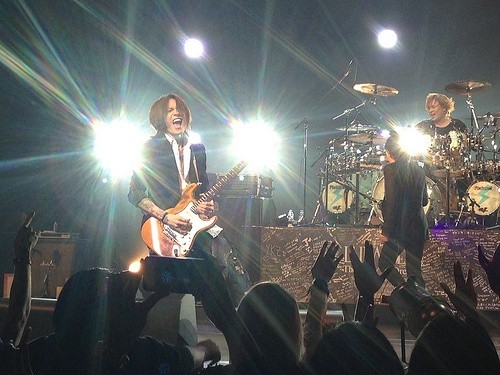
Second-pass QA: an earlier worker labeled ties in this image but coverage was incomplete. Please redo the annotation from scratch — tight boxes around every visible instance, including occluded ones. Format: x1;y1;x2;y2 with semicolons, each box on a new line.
177;140;184;193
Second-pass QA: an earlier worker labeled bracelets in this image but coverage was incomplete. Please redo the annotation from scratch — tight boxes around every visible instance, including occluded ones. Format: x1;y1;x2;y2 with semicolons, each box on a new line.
161;212;169;224
13;255;31;266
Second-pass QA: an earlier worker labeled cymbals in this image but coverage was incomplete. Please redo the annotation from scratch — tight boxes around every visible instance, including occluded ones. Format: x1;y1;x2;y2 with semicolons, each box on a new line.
346;134;386;143
353;83;398;97
445;79;492;95
335;124;380;132
465;111;500;119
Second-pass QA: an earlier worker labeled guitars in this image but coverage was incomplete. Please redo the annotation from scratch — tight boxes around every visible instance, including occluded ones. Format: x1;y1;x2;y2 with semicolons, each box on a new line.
330;177;383;209
140;158;248;257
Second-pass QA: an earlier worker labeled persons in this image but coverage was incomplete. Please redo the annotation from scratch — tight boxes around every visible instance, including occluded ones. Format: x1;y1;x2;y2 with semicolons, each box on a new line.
26;266;222;375
410;93;473;167
0;210;41;375
127;93;250;311
206;234;500;375
376;136;429;292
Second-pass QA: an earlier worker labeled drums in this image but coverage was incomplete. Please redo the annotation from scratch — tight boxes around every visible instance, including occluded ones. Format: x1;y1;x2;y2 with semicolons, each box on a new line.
371;173;452;228
320;180;355;212
463;180;500;216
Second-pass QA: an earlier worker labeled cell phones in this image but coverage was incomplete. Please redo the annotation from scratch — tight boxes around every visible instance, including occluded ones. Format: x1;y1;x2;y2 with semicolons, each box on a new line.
141;256;211;297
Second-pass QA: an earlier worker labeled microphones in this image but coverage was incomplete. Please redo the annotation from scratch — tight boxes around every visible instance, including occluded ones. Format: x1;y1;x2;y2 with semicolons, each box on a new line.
353;59;358;81
